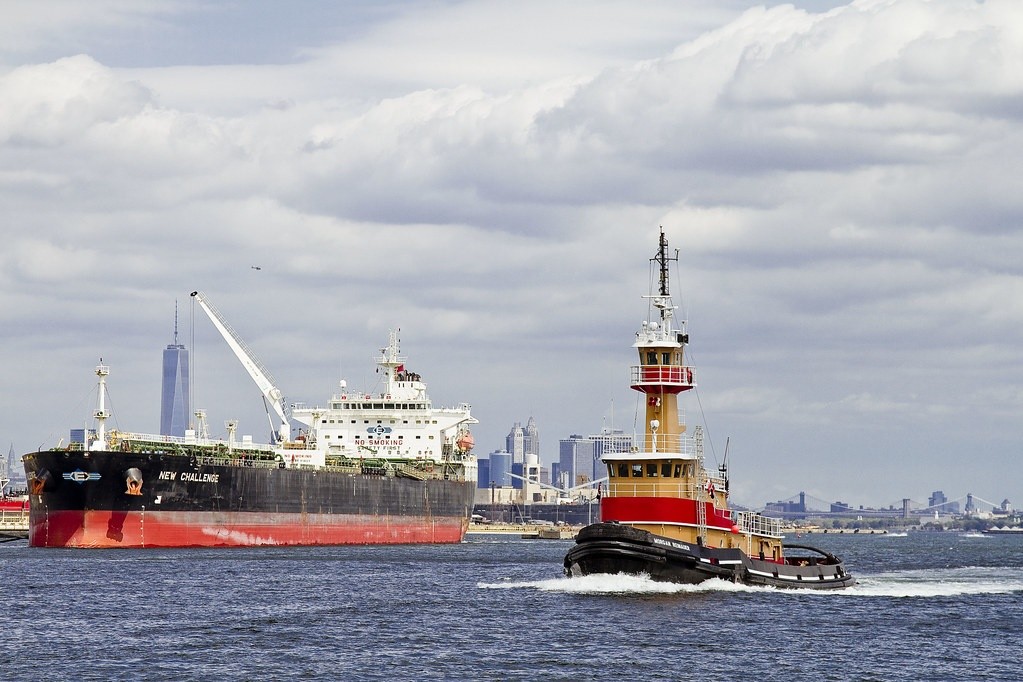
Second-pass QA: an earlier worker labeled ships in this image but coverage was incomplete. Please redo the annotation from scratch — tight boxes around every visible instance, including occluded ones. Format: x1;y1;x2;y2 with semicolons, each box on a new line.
21;291;482;552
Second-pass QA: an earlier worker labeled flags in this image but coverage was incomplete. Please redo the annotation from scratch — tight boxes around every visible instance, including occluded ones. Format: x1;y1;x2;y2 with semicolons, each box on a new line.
397;365;404;372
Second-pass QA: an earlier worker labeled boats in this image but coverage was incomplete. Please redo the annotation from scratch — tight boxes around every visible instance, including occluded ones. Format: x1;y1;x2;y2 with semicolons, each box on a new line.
462;500;588;542
0;476;29;538
563;225;858;588
